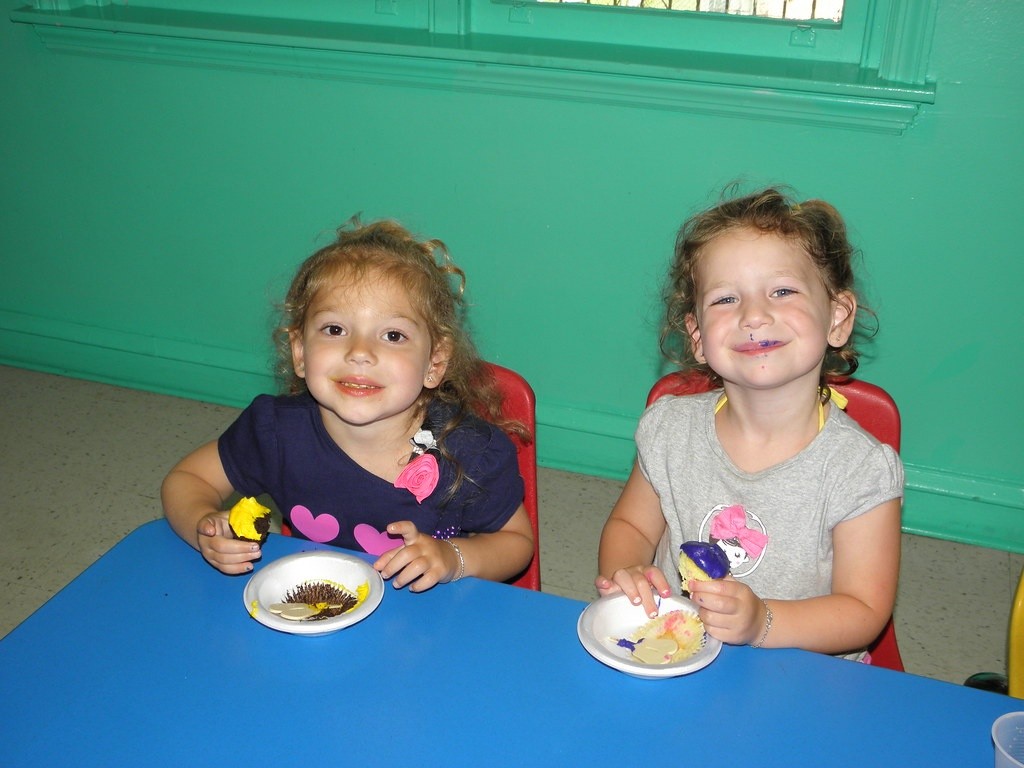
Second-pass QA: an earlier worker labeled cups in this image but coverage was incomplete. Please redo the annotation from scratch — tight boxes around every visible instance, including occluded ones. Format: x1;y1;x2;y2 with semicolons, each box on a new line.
991;711;1024;768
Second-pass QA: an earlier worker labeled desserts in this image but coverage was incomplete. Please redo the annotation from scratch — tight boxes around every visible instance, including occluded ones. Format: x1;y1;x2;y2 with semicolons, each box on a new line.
678;540;730;592
229;496;271;548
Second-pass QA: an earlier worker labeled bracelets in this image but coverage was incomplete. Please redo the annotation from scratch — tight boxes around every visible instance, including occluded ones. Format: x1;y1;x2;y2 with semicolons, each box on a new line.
443;539;464;583
750;598;773;649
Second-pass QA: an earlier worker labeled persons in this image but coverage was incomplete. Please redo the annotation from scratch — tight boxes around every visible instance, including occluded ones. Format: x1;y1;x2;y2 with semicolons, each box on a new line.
590;181;908;667
160;220;536;594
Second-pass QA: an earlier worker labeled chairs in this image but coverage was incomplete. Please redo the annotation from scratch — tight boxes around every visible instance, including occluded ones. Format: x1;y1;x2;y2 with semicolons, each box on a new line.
282;359;542;594
646;364;910;673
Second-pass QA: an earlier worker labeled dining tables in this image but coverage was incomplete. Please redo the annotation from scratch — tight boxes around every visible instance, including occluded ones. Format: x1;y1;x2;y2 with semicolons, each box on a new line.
0;513;1024;767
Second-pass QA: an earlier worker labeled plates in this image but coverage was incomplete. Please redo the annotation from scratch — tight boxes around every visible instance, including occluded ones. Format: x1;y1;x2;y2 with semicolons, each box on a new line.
576;587;723;680
243;550;385;637
